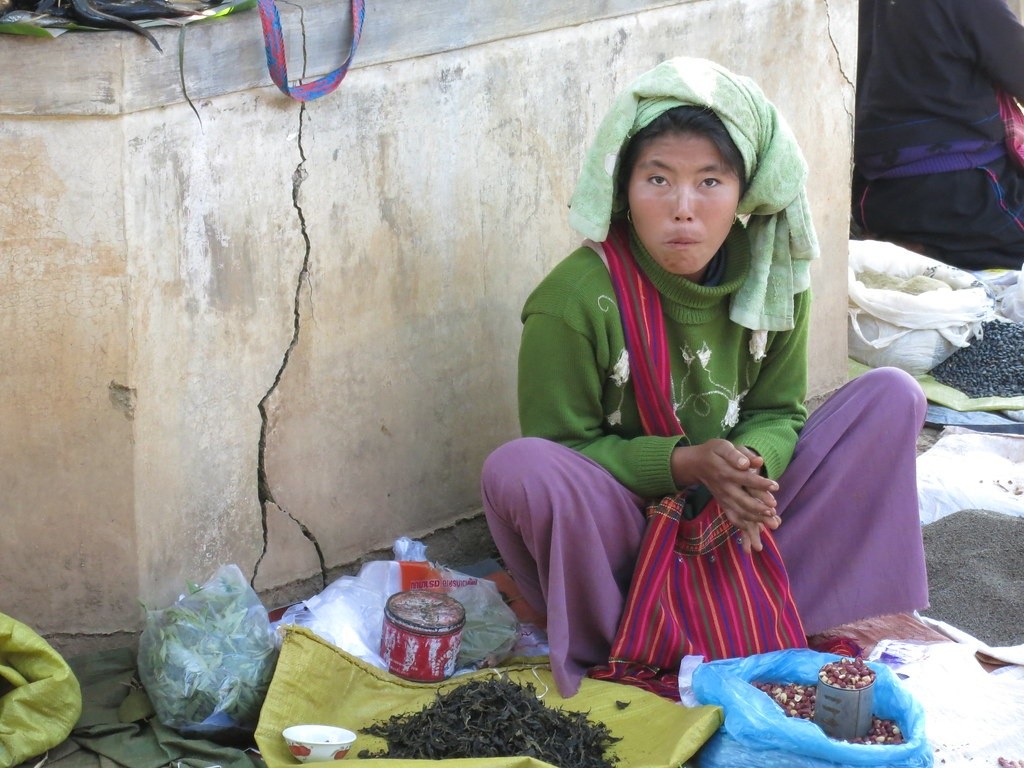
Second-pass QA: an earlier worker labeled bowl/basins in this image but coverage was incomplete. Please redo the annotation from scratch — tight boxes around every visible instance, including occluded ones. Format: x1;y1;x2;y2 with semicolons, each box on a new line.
281;724;356;764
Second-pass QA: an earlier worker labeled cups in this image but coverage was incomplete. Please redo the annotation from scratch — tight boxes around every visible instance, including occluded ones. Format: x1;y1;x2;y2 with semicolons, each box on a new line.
814;662;877;740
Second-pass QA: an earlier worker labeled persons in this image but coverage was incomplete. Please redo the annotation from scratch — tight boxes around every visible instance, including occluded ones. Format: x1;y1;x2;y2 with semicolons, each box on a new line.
857;0;1024;271
480;55;929;700
